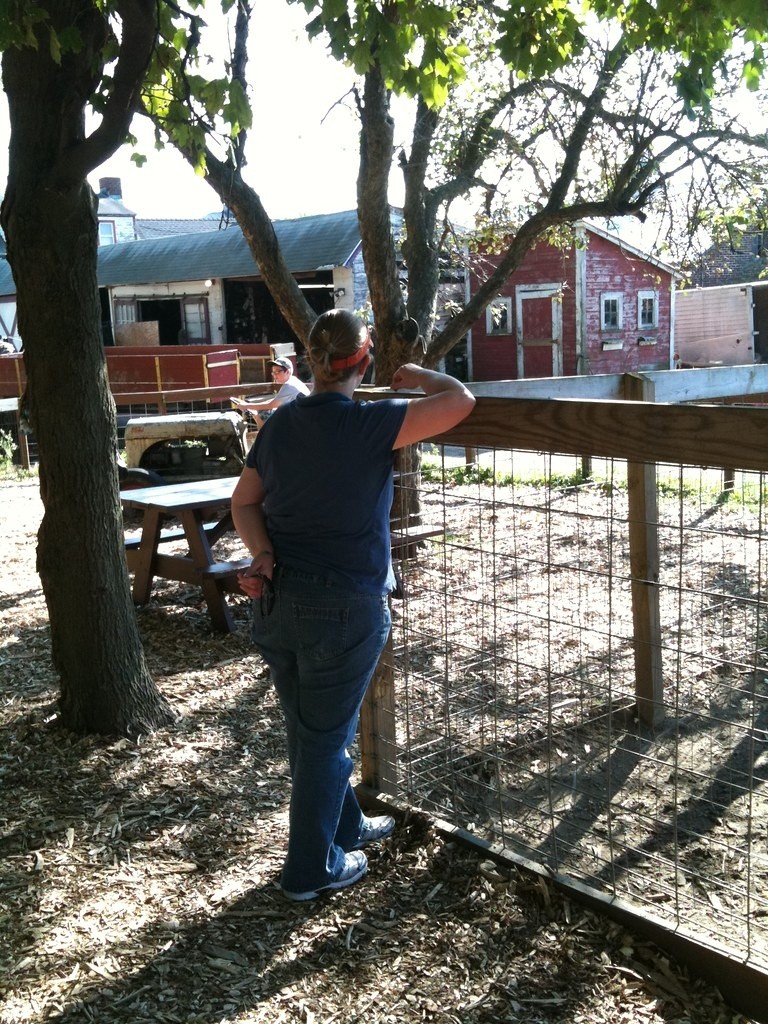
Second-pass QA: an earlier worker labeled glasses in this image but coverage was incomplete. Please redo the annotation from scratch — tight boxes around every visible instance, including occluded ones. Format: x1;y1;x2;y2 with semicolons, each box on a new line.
270;369;284;376
253;573;275;619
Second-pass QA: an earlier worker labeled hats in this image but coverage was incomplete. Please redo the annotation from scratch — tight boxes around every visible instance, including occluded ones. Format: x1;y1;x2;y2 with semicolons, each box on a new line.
266;357;294;376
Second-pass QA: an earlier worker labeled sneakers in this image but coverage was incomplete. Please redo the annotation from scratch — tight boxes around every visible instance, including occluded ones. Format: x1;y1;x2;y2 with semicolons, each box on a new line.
282;850;369;900
352;809;396;849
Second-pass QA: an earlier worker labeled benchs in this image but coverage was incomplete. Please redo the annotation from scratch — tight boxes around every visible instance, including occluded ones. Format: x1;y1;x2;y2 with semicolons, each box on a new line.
204;523;445;600
120;474;242;632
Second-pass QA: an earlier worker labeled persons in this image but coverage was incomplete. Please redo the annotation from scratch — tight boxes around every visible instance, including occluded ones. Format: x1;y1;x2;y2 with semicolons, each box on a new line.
231;306;477;902
235;357;311;430
